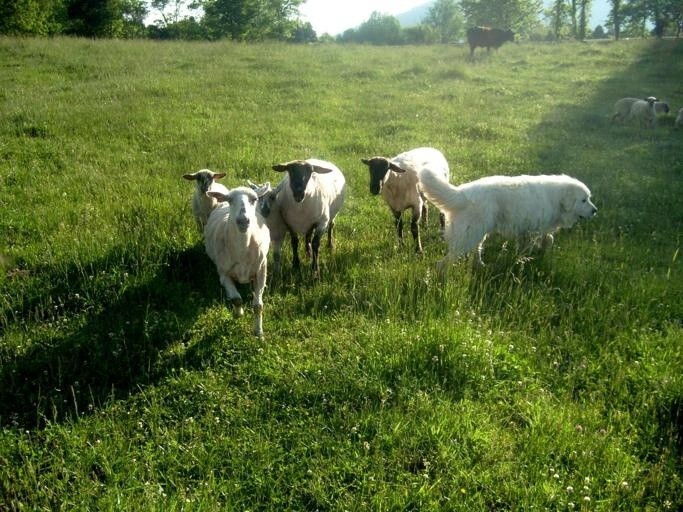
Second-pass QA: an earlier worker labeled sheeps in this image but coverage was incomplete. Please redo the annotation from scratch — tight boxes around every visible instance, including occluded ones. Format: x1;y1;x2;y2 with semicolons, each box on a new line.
361;146;450;262
612;98;683;129
203;182;271;336
271;157;346;282
240;178;286;273
183;169;231;234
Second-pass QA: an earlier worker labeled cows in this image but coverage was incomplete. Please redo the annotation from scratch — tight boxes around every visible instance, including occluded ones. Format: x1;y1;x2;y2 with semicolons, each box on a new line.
466;26;516;57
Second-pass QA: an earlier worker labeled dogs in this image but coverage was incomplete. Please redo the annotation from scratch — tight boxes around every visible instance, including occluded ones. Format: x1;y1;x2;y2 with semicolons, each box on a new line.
417;166;598;277
